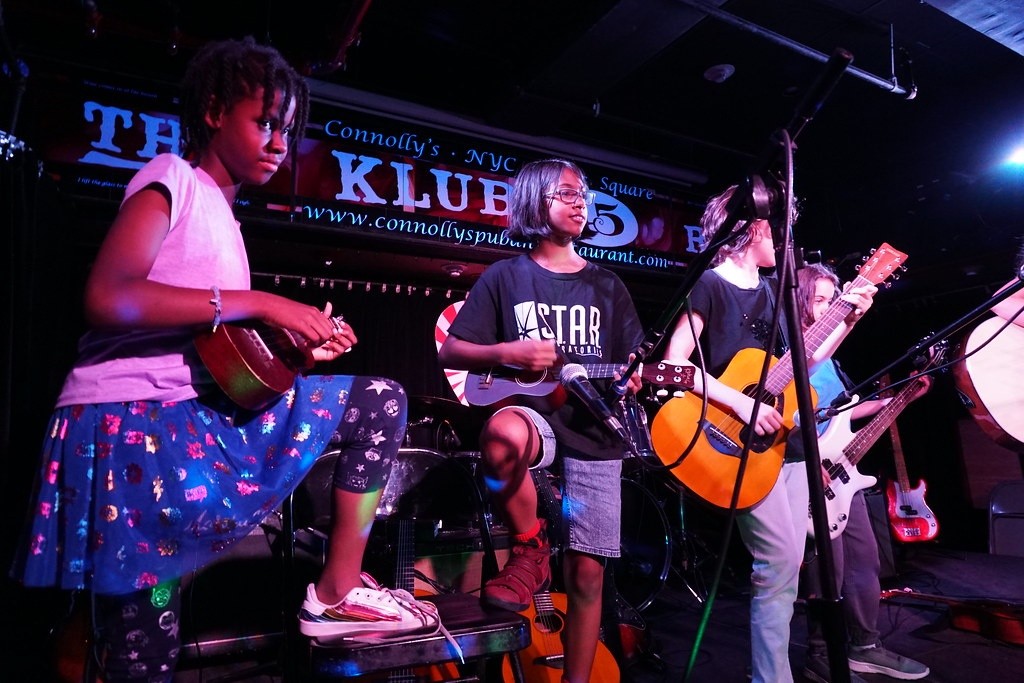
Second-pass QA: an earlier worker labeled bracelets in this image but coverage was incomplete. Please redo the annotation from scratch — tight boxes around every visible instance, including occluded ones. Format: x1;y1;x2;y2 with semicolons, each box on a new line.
208;286;222;332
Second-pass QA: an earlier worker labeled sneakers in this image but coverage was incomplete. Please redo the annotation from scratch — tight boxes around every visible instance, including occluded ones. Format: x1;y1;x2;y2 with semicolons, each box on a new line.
485;539;552;611
803;652;867;683
848;639;930;680
300;572;465;664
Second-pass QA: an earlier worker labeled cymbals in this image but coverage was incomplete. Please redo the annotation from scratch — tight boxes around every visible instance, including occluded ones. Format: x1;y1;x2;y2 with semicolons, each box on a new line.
412;392;489;429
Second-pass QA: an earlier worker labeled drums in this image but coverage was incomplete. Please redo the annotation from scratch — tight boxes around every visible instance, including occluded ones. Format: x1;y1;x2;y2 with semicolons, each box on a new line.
567;465;711;622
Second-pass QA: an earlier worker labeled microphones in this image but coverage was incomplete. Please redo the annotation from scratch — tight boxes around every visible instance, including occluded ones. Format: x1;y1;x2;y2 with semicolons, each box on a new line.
559;362;629;443
794;407;838;427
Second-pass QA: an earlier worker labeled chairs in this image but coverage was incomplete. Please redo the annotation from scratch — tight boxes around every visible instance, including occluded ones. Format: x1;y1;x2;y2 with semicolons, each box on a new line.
287;444;536;683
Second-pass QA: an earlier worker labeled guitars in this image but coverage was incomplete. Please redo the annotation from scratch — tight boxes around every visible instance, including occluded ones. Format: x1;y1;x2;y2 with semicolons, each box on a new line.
808;323;959;540
880;587;1024;645
195;306;348;416
488;446;623;683
950;312;1024;452
464;345;697;419
873;373;942;545
359;486;469;683
643;239;909;513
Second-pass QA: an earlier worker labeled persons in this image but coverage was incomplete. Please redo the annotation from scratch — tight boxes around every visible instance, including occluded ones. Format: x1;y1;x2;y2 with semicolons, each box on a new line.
665;176;812;683
792;265;931;680
22;37;442;683
437;157;645;683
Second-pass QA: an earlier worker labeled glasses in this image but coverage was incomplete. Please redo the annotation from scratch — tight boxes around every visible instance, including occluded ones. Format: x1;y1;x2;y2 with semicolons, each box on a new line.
544;188;596;206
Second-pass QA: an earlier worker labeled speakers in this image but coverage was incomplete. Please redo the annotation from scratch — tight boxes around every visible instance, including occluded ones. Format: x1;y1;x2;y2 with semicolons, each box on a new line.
864;494;895;580
